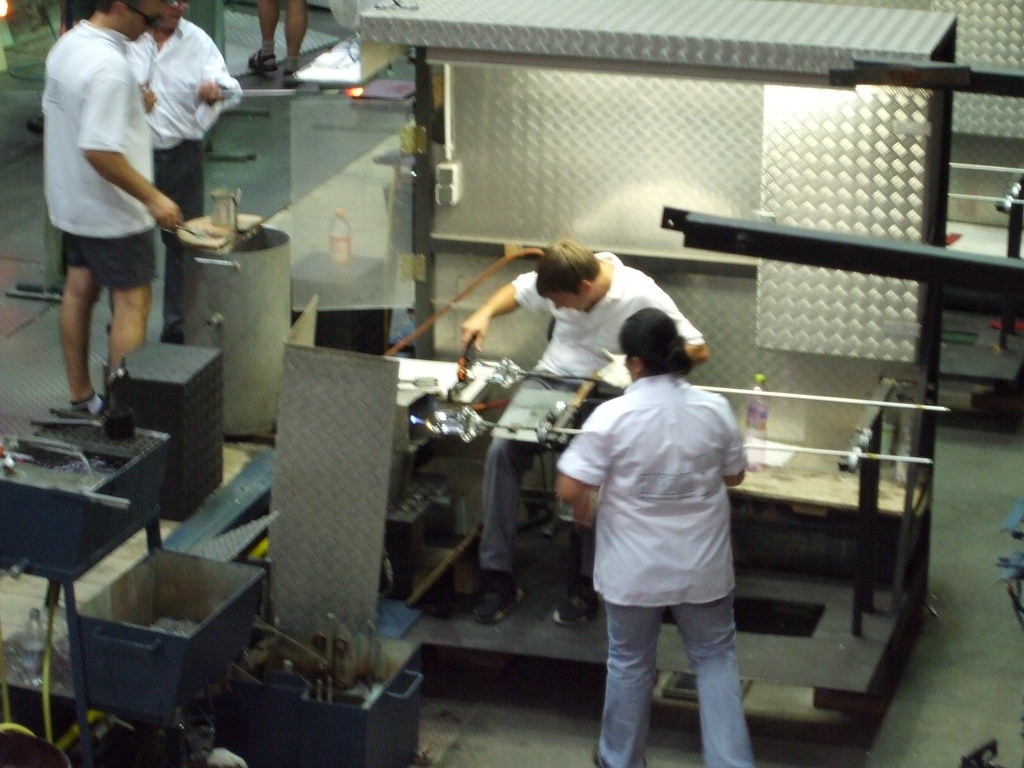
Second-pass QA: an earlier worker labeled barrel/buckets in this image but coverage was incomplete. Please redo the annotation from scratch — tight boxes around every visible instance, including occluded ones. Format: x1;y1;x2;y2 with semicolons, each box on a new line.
164;226;292;435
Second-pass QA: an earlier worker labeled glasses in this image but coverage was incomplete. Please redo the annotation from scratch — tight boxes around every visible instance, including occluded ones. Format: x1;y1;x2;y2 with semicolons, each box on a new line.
127;5;159;28
162;0;187;9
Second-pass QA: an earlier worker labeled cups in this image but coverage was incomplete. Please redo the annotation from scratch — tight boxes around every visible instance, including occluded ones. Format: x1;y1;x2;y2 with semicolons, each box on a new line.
212;188;239;227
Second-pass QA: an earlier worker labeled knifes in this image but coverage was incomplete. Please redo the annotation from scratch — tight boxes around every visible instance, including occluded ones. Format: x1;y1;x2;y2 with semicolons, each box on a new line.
177;222;210;239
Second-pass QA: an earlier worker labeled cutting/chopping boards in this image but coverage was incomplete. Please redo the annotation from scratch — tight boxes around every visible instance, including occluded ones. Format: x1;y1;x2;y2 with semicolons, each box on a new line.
176;213;262;252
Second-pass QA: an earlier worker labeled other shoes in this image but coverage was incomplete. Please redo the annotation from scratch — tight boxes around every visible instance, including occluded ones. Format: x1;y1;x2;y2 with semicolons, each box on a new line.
70;393;108;417
161;333;184;344
594;749;646;768
552;576;599;627
474;571;523;623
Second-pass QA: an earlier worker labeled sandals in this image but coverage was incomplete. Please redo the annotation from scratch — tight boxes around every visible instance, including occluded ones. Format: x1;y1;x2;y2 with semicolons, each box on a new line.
249;49;277;72
283;68;298;87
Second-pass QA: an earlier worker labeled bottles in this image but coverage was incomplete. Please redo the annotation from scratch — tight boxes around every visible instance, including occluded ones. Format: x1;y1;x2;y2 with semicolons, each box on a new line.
328;208;350;266
880;378;899;455
20;609;47;686
744;374;768;471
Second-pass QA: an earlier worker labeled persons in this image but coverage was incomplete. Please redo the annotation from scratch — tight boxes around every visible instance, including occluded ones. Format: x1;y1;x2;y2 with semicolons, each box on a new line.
555;308;756;768
249;1;309;86
42;0;184;413
107;0;242;344
461;241;709;625
